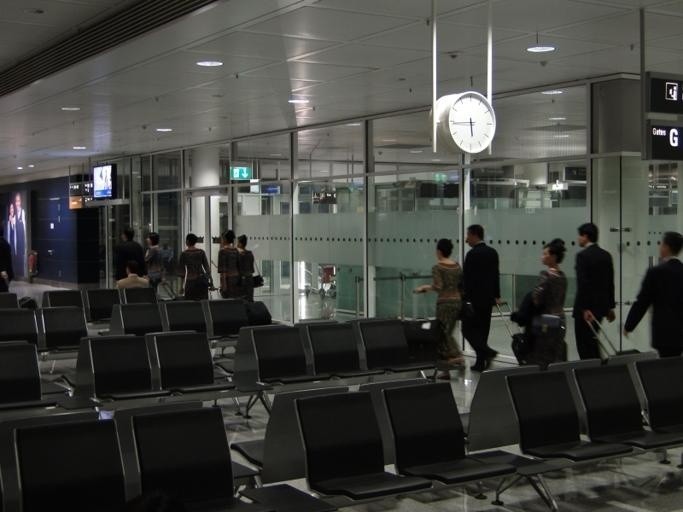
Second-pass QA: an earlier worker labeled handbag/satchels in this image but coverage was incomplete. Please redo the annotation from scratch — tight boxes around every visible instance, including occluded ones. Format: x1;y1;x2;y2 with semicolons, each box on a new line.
531;313;561;338
252;274;264;289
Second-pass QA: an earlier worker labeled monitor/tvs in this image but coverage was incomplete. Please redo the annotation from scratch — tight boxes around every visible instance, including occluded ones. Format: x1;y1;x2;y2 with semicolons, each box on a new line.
92;163;117;199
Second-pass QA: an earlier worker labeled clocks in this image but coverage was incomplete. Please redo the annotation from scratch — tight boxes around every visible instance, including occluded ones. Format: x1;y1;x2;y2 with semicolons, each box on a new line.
429;0;496;158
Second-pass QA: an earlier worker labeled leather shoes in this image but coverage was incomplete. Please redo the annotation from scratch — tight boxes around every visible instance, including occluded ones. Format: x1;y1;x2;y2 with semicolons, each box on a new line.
437;374;449;379
470;350;499;372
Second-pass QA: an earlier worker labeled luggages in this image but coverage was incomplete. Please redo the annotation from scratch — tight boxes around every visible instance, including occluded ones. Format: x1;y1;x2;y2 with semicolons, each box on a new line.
245;300;272;326
161;282;185;300
496;301;530;366
586;315;641;356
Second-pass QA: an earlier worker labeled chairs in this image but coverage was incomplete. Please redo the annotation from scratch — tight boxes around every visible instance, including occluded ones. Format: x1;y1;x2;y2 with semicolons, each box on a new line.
0;286;250;374
0;316;439;421
1;352;683;511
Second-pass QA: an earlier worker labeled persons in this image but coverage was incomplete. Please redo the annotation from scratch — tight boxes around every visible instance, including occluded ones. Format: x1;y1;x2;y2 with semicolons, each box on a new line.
114;226;255;302
6;191;27;277
0;225;15;292
